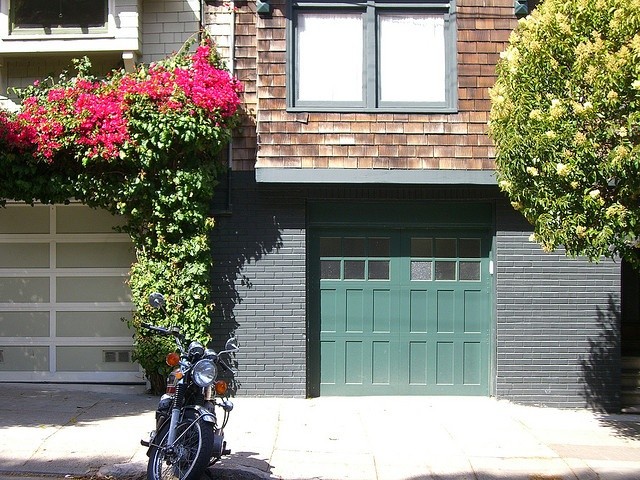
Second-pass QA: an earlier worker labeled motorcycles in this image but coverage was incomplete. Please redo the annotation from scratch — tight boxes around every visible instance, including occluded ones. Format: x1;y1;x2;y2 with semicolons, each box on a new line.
140;292;240;479
